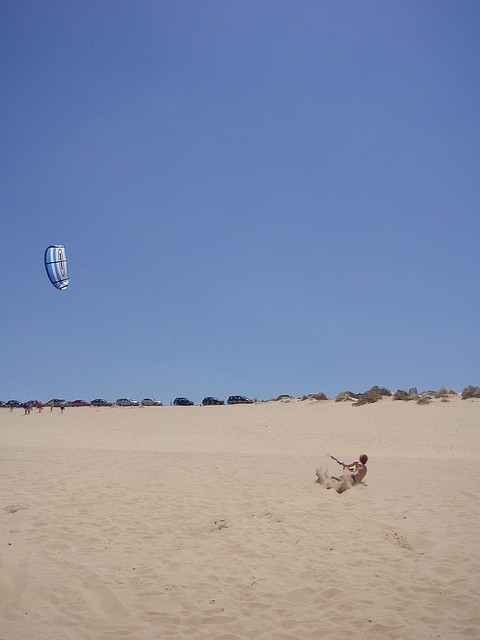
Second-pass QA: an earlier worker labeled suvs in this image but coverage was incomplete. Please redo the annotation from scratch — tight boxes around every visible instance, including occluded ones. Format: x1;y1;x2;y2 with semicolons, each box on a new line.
141;398;162;406
227;396;252;404
173;398;194;406
116;398;139;406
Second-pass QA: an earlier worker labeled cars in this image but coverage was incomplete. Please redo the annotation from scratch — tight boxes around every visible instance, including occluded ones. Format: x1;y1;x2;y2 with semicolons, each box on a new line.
71;400;90;407
46;399;66;407
6;400;22;407
23;400;44;407
202;397;222;405
91;399;113;406
0;401;6;406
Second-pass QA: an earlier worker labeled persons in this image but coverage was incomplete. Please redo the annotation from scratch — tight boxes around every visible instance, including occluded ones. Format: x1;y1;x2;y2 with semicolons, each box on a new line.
334;454;368;492
